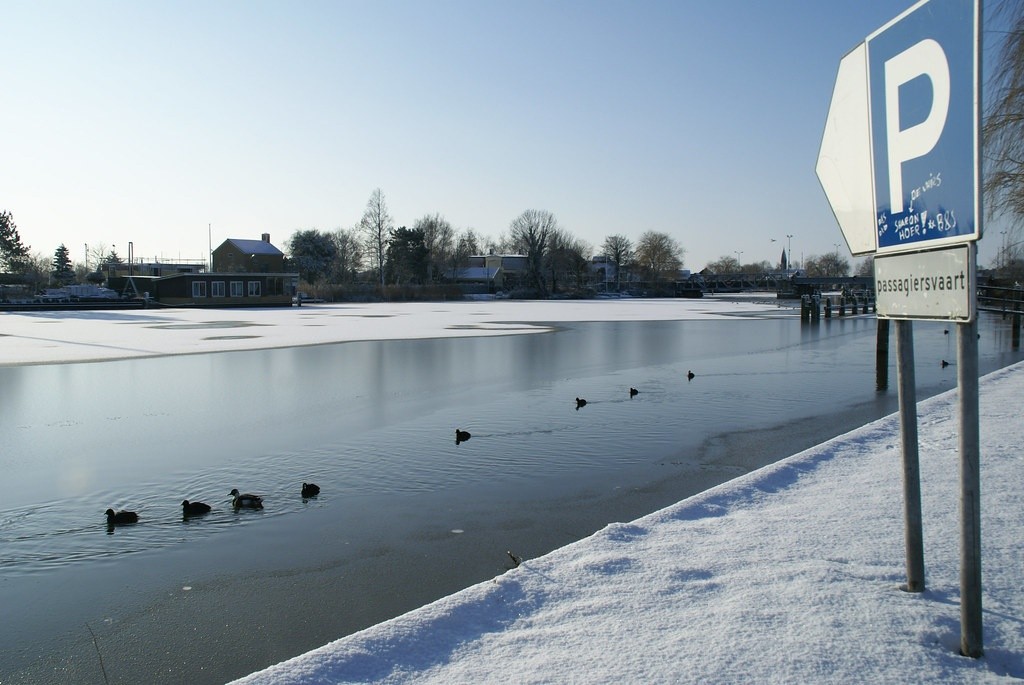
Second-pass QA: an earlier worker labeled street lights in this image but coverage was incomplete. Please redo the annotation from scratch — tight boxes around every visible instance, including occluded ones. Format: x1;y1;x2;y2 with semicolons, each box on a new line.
834;243;840;277
1000;231;1007;268
734;250;743;273
787;234;793;279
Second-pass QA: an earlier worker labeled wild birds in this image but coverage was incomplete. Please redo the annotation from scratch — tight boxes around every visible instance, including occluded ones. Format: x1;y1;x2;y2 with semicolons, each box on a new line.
227;489;264;508
943;329;949;335
455;438;470;445
455;429;471;438
301;482;320;498
688;369;695;376
940;359;949;365
180;500;211;516
104;509;138;524
630;393;638;399
630;387;638;393
575;396;587;404
575;404;586;411
688;376;694;382
